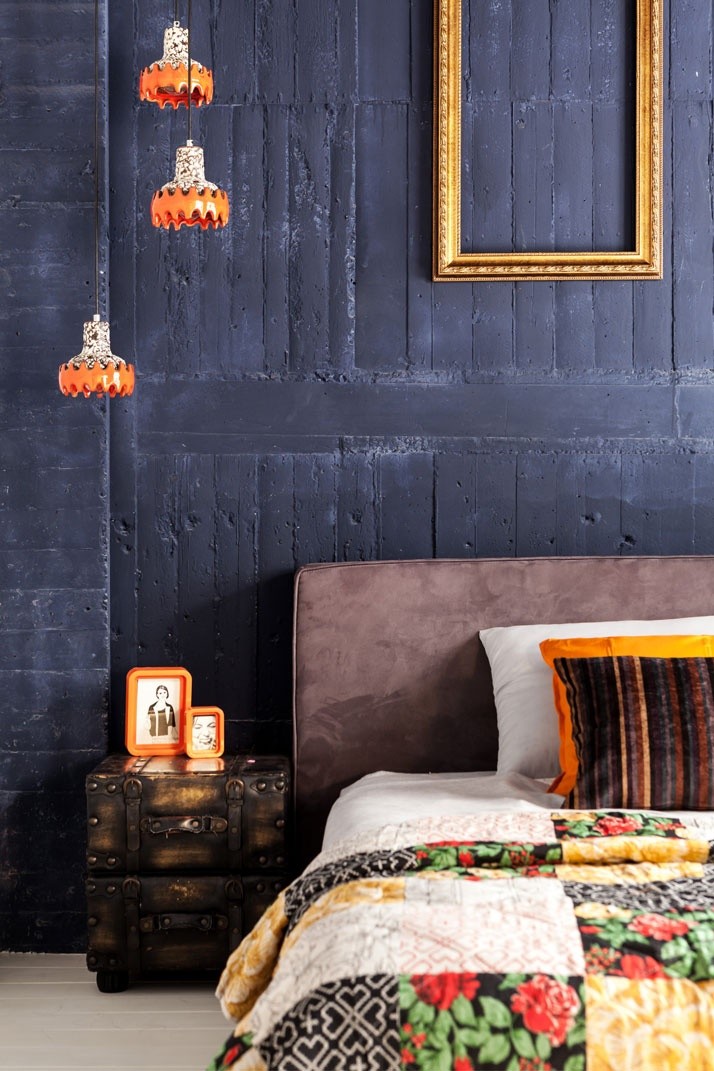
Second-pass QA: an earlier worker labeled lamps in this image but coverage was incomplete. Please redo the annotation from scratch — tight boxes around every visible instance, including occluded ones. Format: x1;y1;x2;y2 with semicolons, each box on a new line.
139;0;213;111
150;0;231;231
57;1;136;400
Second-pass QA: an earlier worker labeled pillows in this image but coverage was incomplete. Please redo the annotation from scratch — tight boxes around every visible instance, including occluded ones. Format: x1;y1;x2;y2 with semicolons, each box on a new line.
476;614;714;780
538;633;714;801
552;654;714;812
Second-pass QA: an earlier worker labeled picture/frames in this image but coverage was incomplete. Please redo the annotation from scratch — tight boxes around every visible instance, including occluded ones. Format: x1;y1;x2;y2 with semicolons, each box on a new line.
125;666;193;757
183;706;226;758
430;0;665;283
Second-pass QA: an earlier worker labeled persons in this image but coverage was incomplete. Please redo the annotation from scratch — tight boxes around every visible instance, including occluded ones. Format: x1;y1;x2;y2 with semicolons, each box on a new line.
142;685;178;744
193;716;216;750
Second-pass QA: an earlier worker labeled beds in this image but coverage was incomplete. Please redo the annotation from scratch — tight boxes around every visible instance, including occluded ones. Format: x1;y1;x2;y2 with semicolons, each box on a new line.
209;552;714;1071
